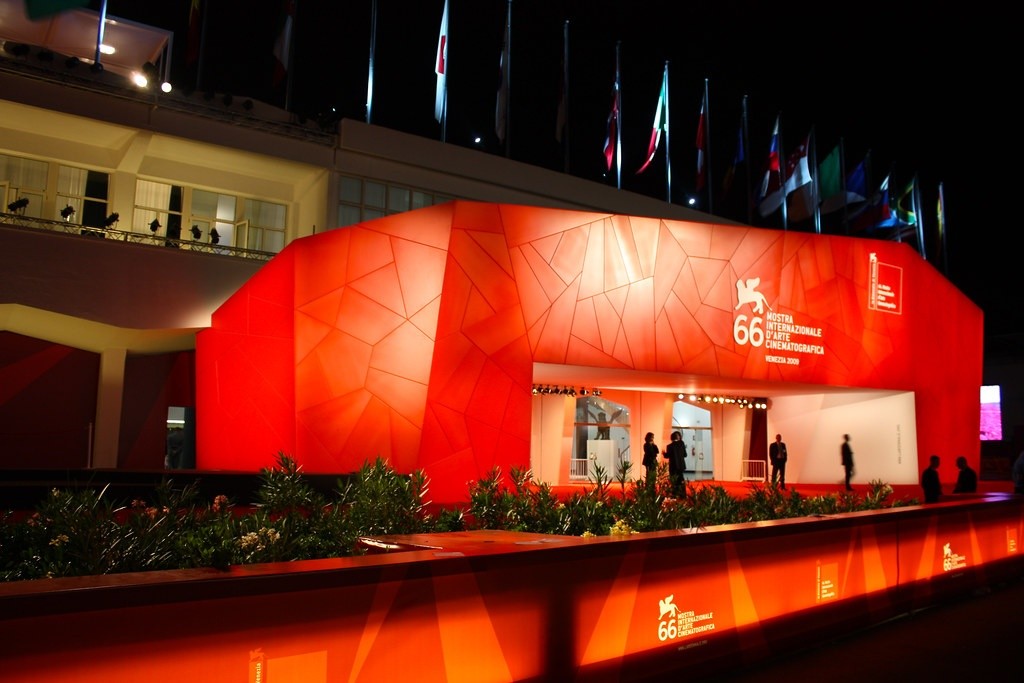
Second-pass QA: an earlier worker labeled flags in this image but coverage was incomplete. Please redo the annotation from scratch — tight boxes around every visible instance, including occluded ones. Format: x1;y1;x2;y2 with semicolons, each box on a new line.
187;0;200;64
695;97;944;263
496;19;508;143
602;73;619;172
434;0;449;122
635;70;665;174
555;60;565;143
23;0;91;20
272;0;296;87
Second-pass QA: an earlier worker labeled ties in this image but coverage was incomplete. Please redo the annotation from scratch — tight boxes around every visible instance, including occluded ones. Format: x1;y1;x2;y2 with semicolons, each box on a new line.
778;443;782;456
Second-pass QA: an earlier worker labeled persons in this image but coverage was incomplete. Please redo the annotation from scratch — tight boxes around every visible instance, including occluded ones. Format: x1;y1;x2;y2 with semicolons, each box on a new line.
662;432;687;499
953;458;977;493
1012;452;1024;493
841;435;853;490
642;432;659;489
921;455;943;503
166;427;184;469
769;434;787;489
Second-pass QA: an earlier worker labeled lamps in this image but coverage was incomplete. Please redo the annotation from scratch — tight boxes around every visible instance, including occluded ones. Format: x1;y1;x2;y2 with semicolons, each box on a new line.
8;198;30;213
208;227;222;243
533;384;601;397
678;393;768;409
189;224;202;240
149;218;162;233
61;205;75;220
103;212;119;228
